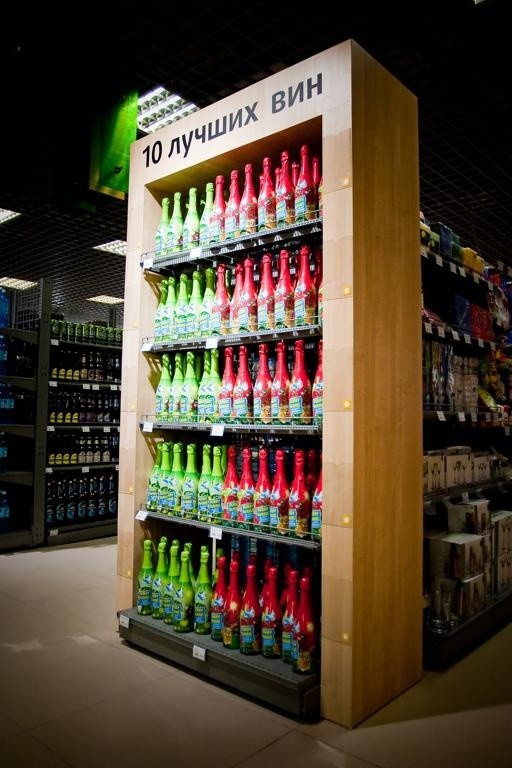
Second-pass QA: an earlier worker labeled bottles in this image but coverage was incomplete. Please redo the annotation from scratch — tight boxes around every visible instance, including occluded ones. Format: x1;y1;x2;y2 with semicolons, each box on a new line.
182;188;199;250
102;437;110;461
51;350;58;378
88;325;95;338
73;351;79;379
237;258;258;332
0;432;8;472
211;264;231;334
174;274;189;339
210;557;227;642
292;576;315;675
163;546;180;624
156;443;171;513
305;449;316;493
71;395;79;422
50;319;59;336
155;198;170;257
239;164;257;237
311;339;323;424
211;549;224;588
62;435;70;464
81;324;88;338
293;246;316;326
74;323;81;337
59;321;66;337
95;326;102;339
79;394;85;422
224;170;238;241
208;446;225;523
210;176;224;243
70;435;78;463
168;442;185;517
102;328;108;339
57;394;63;422
97;394;104;422
199;183;214;246
254;448;272;533
318;173;323;218
289;450;310;539
161;536;169;572
0;335;8;375
104;395;111;423
161;276;177;342
67;322;74;337
313;252;321;286
114;328;121;341
166;192;182;253
240;565;261;655
172;540;181;577
86;437;94;463
186;271;203;338
88;352;94;380
180;352;198;422
221;448;240;526
168;354;184;422
261;568;280;658
275;151;294;227
233;346;254;424
154;354;171;421
5;384;14;423
95;353;102;379
229;263;243;332
152;542;168;618
270;448;290;536
86;395;93;421
274;250;294;328
312;155;319;186
197;350;211;423
288;340;311;423
80;354;87;379
198;445;212;522
257;158;276;230
254;344;272;424
66;350;73;378
291;162;299;187
137;540;154;615
153;279;168;341
50;394;56;423
199;268;216;337
317;279;324;325
281;571;299;664
237;447;256;530
64;394;71;422
204;348;221;423
112;395;119;423
270;342;291;424
48;435;55;464
257;254;275;329
55;435;62;464
294;144;314;221
94;437;100;462
223;562;241;648
145;441;164;511
221;445;227;479
181;444;200;519
108;327;114;340
174;551;195;633
194;553;213;634
78;437;85;463
185;543;196;587
0;383;4;424
58;350;66;378
114;355;120;382
0;491;10;534
218;347;235;423
310;454;322;540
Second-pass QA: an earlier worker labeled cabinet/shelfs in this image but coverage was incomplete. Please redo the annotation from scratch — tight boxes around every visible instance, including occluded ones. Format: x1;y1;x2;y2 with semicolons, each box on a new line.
117;38;424;729
419;220;512;673
49;278;123;546
0;277;48;550
